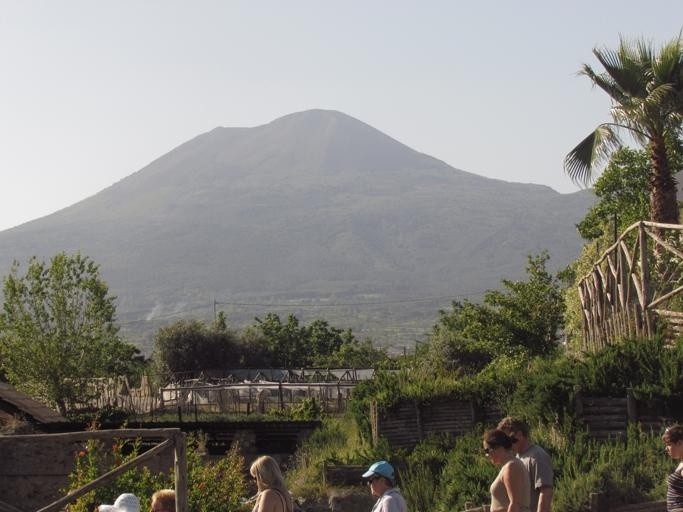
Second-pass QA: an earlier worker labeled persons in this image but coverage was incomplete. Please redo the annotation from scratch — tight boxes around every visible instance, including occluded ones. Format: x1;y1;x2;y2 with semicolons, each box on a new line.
248;454;293;511
661;423;683;511
149;489;176;511
494;415;555;511
482;429;534;512
359;459;408;512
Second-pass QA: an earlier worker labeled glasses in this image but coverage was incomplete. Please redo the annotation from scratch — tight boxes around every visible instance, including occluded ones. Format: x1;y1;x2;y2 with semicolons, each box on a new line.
366;477;380;485
483;447;493;453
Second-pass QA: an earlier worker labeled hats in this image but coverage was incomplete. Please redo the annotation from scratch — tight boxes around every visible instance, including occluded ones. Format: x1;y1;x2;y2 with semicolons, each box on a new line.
360;460;394;479
